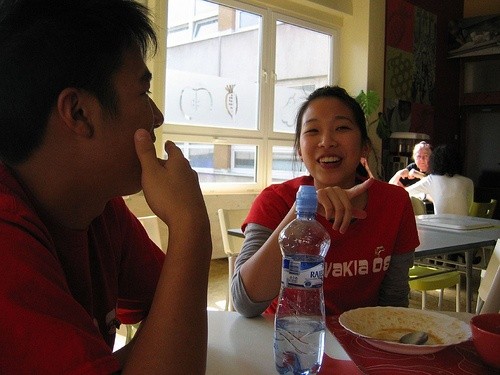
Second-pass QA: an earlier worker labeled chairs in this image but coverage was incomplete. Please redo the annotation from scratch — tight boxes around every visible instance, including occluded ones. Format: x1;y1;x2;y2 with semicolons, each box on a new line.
123;200;500;346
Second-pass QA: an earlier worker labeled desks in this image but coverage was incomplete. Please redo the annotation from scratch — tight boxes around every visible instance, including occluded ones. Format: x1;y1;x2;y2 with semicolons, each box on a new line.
206;309;500;375
227;212;500;313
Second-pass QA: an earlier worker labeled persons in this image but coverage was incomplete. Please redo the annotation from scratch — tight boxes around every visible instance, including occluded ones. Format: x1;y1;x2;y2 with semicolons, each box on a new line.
0;0;221;375
231;86;420;318
390;142;434;216
401;147;481;275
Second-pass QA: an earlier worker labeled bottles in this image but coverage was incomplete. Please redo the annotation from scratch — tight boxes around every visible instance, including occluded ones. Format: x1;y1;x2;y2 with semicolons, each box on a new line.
272;185;331;375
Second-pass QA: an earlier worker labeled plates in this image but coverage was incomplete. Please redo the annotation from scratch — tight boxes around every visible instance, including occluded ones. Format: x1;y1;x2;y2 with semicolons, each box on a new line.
338;307;472;356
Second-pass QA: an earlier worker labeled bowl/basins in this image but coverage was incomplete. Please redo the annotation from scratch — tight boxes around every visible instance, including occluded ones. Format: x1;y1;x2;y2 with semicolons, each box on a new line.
470;312;500;370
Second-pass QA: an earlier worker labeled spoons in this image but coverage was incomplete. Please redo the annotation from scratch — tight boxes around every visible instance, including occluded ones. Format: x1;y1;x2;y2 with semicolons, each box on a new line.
359;332;429;345
